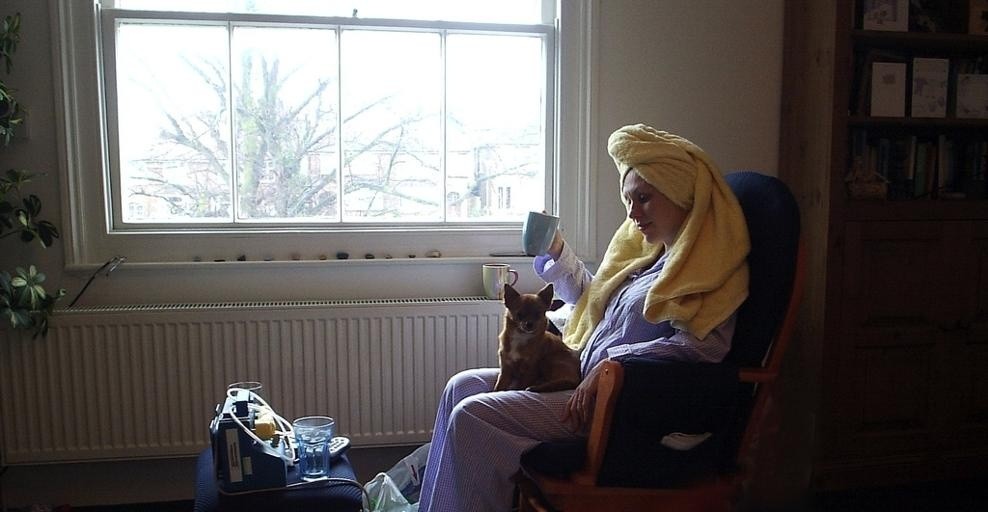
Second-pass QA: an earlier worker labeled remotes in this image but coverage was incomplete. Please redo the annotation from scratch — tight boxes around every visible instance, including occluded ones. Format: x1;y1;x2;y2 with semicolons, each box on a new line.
328;437;351;461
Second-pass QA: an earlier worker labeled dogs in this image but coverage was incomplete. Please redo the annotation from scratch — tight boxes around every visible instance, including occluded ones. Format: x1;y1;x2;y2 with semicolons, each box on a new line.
493;283;581;393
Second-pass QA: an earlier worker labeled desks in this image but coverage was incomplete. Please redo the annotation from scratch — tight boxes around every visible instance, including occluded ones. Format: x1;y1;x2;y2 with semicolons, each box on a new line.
194;445;365;511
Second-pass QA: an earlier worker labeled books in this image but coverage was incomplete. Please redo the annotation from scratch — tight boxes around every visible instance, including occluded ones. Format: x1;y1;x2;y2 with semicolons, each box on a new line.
853;1;987;201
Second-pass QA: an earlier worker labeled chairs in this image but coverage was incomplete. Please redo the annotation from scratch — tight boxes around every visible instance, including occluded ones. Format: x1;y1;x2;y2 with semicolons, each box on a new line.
512;171;807;512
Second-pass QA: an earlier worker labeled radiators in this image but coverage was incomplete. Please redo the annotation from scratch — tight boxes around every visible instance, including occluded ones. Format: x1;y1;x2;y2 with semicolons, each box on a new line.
0;295;510;466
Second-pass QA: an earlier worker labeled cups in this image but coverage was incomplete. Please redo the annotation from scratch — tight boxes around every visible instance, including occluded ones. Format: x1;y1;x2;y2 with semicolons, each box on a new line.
483;262;520;302
291;414;334;482
228;381;262;397
521;211;561;258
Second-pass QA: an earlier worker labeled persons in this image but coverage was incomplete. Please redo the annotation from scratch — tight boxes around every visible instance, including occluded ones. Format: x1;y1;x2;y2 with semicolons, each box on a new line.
417;122;753;512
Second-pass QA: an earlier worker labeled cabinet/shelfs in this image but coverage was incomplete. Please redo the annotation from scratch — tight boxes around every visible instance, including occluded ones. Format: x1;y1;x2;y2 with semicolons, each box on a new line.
776;0;988;501
